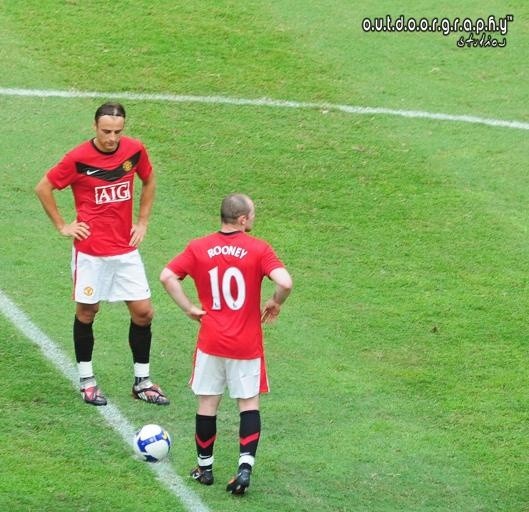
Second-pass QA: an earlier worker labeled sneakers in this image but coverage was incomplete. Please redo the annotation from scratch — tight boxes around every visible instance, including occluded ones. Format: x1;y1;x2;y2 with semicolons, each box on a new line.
226;468;250;495
133;384;170;404
80;384;107;405
191;467;213;485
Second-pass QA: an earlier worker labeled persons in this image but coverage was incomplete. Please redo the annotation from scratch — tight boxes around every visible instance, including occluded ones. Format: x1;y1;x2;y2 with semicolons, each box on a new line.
35;104;169;406
161;194;292;494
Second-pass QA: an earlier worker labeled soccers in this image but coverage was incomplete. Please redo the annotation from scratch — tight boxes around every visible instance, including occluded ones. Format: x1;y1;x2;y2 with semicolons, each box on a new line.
134;424;170;463
